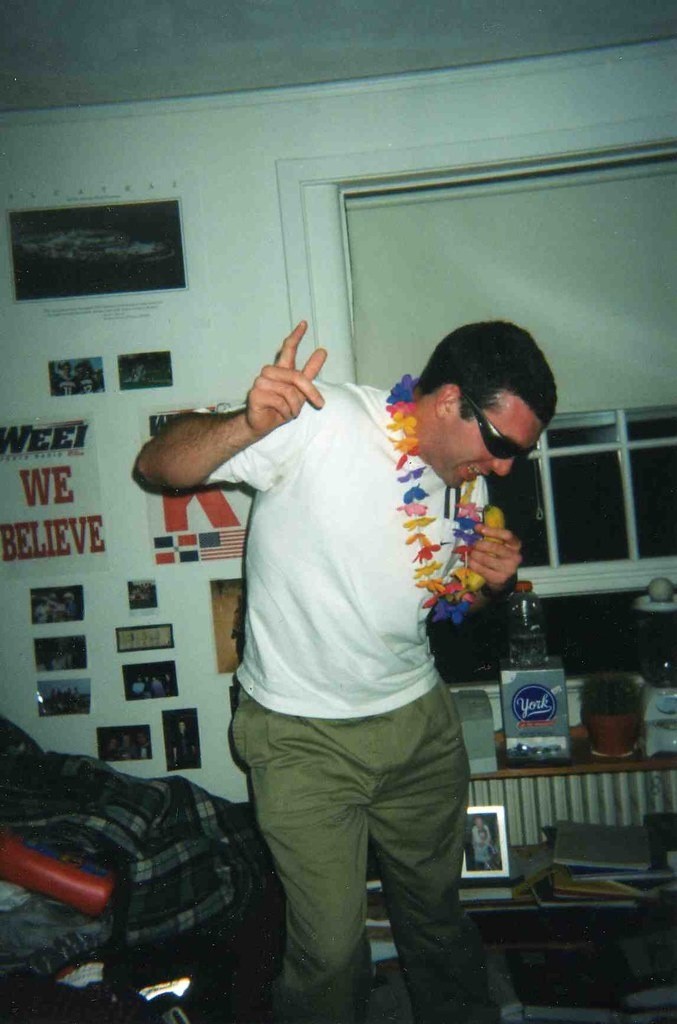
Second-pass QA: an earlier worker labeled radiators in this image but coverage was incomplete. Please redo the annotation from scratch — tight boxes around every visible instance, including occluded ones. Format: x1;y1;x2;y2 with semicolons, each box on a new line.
465;769;677;847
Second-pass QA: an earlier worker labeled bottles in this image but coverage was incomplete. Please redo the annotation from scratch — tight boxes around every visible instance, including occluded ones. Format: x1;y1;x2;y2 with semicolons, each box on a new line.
508;580;550;669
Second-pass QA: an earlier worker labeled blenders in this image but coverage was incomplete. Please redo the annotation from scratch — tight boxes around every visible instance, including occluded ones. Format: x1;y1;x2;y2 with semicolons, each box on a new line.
633;579;677;760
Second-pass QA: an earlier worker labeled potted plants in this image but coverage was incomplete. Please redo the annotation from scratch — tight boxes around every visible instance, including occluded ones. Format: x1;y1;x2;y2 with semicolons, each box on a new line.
581;682;642;756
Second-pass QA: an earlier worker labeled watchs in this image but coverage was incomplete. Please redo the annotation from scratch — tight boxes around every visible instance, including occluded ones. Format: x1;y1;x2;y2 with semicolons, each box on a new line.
482;574;518;601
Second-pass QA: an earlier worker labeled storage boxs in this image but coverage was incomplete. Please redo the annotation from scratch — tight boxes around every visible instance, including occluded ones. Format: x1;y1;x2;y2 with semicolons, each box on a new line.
499;656;574;765
452;689;498;775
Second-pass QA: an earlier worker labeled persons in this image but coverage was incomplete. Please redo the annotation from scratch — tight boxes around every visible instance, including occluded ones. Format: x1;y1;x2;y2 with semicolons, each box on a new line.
54;360;98;396
133;672;176;699
132;583;153;601
50;687;80;715
108;732;152;761
50;643;73;671
171;718;196;770
137;320;558;1024
33;592;78;624
472;815;496;870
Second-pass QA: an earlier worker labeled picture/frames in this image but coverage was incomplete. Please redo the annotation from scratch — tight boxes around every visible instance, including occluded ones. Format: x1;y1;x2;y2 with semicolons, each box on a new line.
460;805;510;878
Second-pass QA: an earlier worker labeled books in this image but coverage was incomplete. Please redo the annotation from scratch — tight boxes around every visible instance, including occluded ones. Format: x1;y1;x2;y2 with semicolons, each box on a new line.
514;813;677;907
496;962;677;1024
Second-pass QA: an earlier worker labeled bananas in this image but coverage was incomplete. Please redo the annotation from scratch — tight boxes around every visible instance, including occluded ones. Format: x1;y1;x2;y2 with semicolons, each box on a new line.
465;504;505;592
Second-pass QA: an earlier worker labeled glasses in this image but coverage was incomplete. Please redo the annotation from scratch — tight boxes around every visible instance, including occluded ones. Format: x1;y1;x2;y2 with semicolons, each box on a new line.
461;390;537;461
